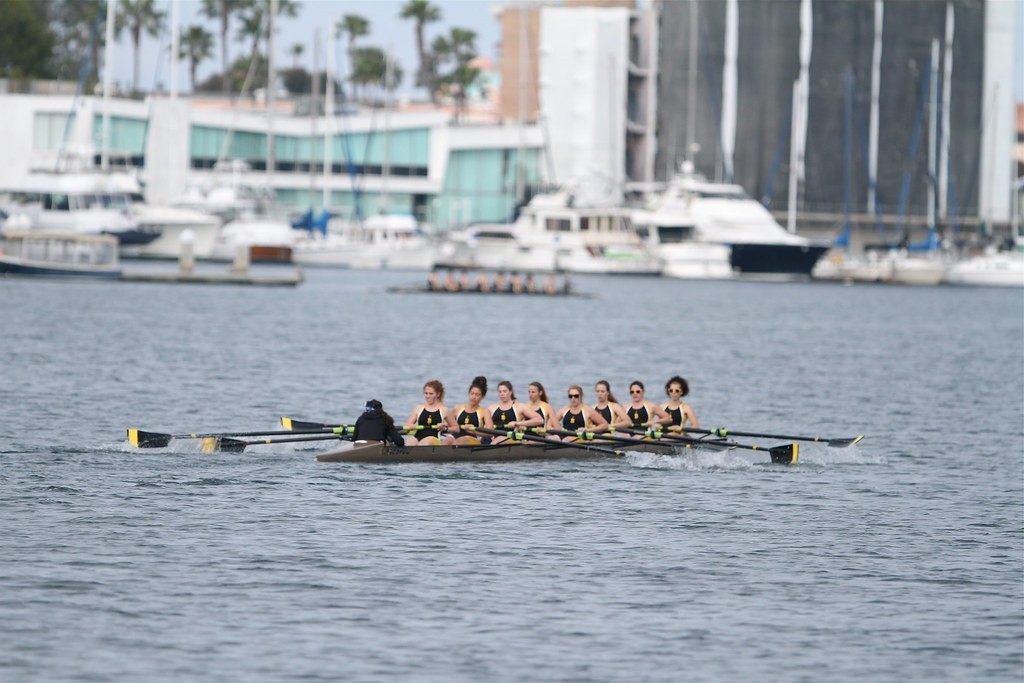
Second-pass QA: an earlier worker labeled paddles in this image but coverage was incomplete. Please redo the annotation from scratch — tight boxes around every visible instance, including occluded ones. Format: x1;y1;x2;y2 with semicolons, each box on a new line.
458;425;627;458
660;425;864;448
127;428;356;455
201;434;355;453
607;425;799;465
281;416;447;431
494;425;692;449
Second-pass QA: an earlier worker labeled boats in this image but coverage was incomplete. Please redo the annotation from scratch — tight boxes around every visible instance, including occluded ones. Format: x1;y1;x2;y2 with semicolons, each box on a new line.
0;230;124;281
316;437;736;464
123;269;306;289
388;284;580;297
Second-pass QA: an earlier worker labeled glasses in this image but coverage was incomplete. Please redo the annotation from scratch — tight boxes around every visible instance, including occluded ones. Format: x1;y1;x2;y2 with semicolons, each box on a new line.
669;388;682;392
569;394;580;398
630;390;642;394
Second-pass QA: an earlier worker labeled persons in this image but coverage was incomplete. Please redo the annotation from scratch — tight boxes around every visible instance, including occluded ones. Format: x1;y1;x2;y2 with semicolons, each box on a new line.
480;381;544;445
427;267;555;295
351;398;404;447
547;384;608;443
519;382;562;444
588;380;633;442
616;380;673;439
402;380;460;445
653;376;699;441
442;377;494;444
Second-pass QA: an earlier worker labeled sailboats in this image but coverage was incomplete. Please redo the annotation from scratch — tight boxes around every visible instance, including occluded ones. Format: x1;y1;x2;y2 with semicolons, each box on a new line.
1;0;1023;286
